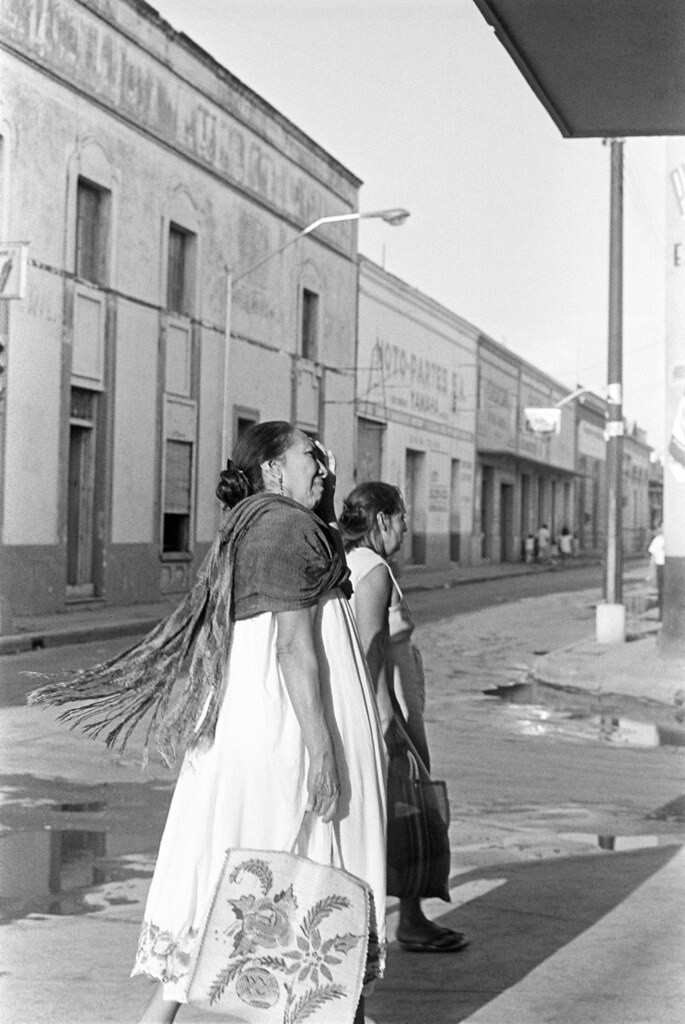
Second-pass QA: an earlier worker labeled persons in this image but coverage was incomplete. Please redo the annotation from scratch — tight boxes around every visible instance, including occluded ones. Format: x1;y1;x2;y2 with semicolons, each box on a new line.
525;522;576;567
333;483;474;954
129;421;389;1023
647;521;664;620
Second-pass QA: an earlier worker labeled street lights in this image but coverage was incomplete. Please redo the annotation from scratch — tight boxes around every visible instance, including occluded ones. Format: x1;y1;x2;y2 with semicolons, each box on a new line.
220;209;410;472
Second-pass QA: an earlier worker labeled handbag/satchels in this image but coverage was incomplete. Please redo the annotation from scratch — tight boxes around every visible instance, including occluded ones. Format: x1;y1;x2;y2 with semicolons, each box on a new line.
376;718;453;903
185;806;370;1024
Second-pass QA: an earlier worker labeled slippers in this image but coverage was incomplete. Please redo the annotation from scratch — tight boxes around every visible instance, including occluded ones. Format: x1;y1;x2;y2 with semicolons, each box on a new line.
398;921;468;952
